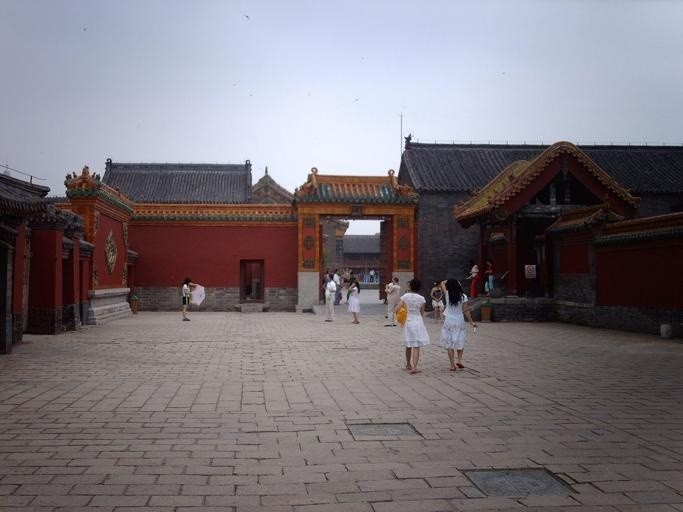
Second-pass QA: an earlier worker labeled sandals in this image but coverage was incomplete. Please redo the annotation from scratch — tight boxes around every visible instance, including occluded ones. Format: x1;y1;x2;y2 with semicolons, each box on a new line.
406;364;422;374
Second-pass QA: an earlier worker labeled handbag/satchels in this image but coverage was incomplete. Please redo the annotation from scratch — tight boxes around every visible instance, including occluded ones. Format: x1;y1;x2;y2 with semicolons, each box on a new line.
396;303;407;324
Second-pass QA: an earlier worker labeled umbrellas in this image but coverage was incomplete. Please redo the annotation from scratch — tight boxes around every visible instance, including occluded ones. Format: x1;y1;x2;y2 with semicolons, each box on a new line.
190;284;206;307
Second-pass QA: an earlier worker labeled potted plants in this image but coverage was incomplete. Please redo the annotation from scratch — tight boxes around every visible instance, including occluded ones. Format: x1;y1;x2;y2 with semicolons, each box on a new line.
129;293;139;315
480;299;493;323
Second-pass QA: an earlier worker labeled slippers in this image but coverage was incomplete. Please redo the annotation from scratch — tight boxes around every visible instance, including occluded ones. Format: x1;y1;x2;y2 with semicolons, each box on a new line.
450;363;464;371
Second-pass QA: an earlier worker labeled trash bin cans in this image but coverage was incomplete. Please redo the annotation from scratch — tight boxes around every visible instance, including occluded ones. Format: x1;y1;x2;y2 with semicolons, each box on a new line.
129;295;138;313
481;300;492;322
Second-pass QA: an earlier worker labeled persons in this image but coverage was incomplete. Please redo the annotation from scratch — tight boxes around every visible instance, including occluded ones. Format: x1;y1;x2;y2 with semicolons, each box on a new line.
322;267;361;324
369;268;376;282
395;279;477;374
182;277;198;321
383;277;401;327
466;258;496;299
